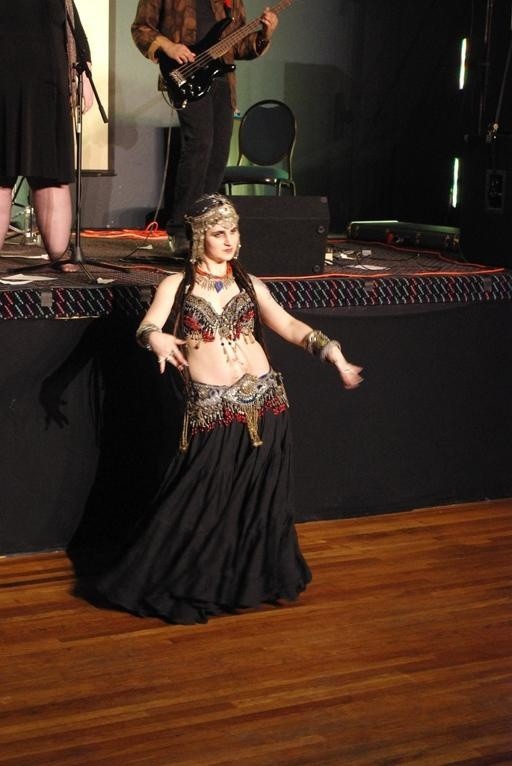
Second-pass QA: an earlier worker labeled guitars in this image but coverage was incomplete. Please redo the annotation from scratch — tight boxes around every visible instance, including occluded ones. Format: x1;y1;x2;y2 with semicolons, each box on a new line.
158;0;293;102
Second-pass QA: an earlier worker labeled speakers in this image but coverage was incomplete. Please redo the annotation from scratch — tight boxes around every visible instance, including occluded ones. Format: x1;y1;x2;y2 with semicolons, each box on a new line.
200;193;331;280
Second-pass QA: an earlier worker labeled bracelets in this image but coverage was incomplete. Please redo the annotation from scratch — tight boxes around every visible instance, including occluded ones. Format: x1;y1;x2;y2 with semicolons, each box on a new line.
303;330;341;361
136;321;162;350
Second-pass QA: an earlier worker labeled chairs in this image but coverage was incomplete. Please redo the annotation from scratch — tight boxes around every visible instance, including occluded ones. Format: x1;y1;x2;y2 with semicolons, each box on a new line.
223;99;297;197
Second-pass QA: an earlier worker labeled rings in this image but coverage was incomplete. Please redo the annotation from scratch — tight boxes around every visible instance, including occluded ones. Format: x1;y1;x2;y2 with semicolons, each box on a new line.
158;351;176;362
342;366;351;373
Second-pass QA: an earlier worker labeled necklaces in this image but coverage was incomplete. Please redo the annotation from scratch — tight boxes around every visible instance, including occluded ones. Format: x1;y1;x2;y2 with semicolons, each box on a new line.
192;272;236;292
194;263;234;278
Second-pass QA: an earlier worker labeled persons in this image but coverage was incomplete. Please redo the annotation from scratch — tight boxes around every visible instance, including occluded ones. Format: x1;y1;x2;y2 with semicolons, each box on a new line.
128;0;280;265
63;190;364;627
1;0;96;276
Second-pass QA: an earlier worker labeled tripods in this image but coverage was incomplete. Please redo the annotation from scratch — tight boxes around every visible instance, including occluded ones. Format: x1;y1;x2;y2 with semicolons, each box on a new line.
8;70;130;283
